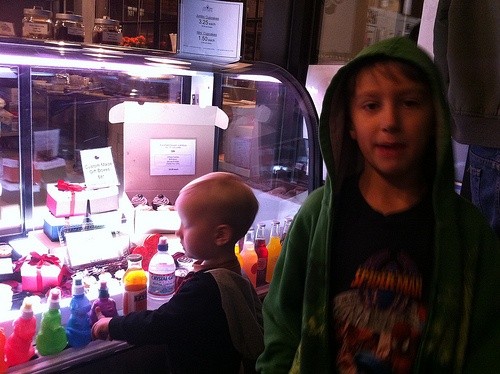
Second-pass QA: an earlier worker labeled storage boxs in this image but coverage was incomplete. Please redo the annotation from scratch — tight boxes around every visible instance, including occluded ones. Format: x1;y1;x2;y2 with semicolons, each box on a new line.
41;101;229;241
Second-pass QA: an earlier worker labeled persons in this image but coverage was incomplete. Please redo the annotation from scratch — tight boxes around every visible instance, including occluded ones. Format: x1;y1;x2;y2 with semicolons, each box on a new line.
433;0;500;233
91;173;266;374
254;39;500;374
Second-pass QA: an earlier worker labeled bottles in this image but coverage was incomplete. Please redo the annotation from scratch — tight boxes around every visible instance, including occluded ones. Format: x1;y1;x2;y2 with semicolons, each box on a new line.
53;11;85;45
21;5;53;40
0;302;37;374
91;15;122;46
36;289;66;356
122;254;147;315
266;217;293;283
255;224;268;287
89;281;120;326
147;237;176;311
65;278;92;348
234;228;259;289
